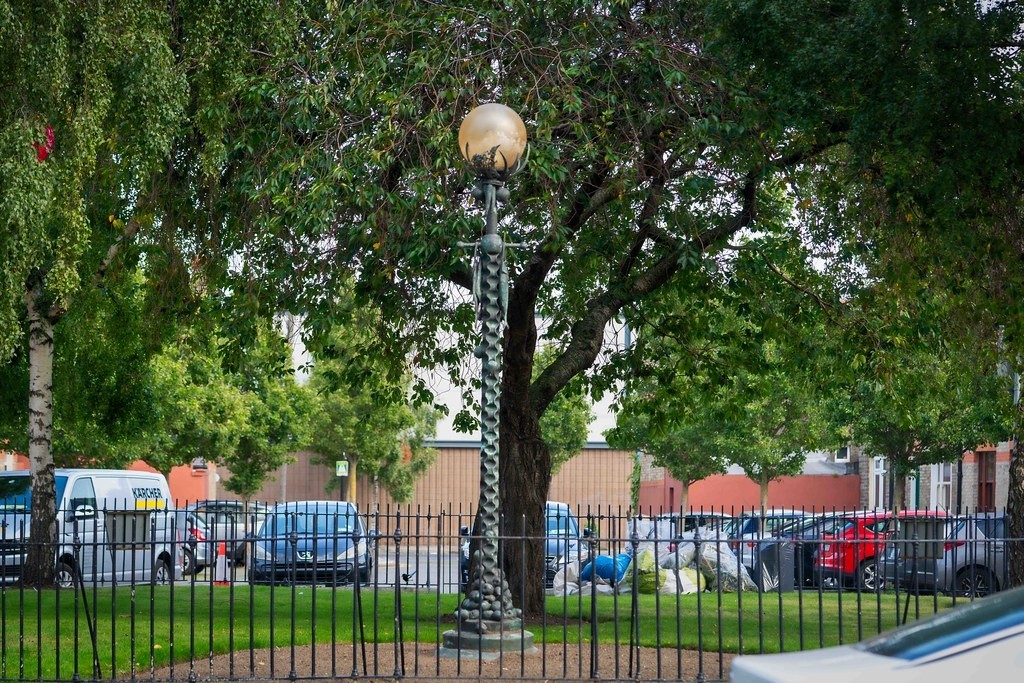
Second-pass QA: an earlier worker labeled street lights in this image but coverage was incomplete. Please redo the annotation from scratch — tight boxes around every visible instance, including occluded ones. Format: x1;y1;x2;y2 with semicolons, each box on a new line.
435;105;537;661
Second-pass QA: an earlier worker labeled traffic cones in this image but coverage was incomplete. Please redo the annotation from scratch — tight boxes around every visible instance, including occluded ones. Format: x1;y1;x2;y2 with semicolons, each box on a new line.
215;542;231;587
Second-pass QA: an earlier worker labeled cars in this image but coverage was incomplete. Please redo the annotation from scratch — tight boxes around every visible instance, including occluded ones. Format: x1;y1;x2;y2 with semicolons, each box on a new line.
878;513;972;594
181;500;275;565
942;512;1010;599
727;584;1024;683
651;505;873;589
242;500;383;587
814;509;955;594
174;509;216;575
459;502;594;595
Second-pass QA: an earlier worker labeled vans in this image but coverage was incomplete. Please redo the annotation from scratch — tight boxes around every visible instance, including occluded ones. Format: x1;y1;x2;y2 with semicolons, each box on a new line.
0;467;184;589
